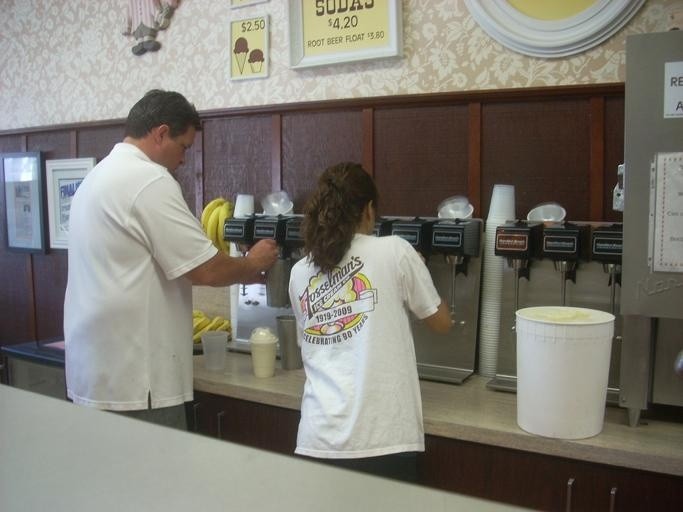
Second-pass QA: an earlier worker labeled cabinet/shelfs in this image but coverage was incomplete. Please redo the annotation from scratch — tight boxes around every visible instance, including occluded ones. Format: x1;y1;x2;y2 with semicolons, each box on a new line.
183;391;681;511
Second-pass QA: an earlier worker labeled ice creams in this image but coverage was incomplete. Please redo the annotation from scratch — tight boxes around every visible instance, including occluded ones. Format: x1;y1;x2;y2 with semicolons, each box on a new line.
248;49;264;73
234;38;249;73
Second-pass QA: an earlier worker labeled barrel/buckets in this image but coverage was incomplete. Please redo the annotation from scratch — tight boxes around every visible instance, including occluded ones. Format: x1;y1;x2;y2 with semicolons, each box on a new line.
516;305;616;440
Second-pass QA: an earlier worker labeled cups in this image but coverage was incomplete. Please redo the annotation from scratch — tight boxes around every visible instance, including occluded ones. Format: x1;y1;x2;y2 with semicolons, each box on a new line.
478;183;516;378
276;315;305;371
202;330;230;374
248;326;279;379
265;257;291;307
233;193;256;218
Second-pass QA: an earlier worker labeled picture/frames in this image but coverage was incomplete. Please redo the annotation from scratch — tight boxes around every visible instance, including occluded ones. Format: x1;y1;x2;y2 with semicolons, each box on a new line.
229;0;404;80
0;151;98;256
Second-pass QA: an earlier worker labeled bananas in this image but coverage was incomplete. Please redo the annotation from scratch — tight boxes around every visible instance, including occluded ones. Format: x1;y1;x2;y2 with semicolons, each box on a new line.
192;310;231;344
201;197;234;255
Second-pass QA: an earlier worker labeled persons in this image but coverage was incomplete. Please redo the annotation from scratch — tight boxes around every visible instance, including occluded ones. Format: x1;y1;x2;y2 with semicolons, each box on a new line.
289;161;453;478
63;89;279;430
